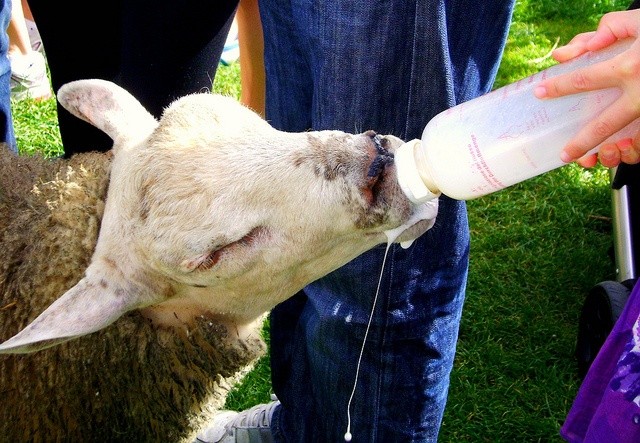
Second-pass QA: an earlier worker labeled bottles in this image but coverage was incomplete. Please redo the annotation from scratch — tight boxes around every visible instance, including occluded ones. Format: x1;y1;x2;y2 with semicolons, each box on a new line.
393;36;640;204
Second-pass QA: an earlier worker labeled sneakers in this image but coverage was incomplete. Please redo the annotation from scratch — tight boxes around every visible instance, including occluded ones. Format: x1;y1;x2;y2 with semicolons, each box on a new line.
192;398;281;442
8;51;52;101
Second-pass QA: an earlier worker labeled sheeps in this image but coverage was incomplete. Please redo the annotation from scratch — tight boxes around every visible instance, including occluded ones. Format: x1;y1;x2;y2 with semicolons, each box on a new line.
0;78;440;443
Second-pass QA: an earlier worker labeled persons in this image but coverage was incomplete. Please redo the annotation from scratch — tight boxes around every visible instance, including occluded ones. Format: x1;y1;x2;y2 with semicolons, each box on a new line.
260;1;515;442
235;0;270;125
21;0;239;159
1;0;28;155
7;0;53;105
534;8;640;168
